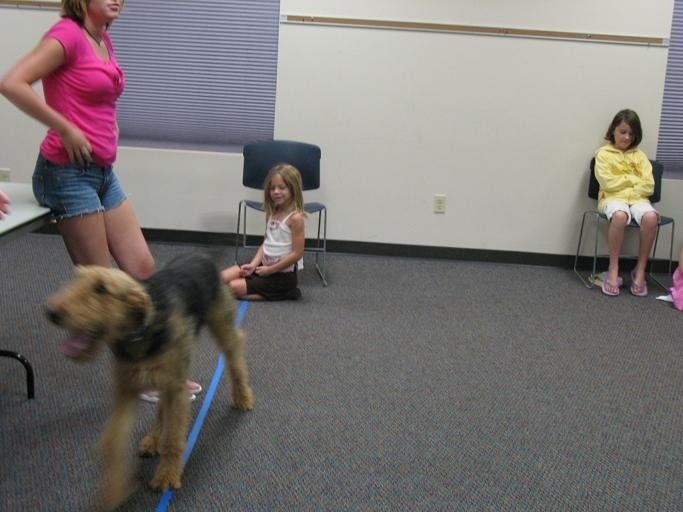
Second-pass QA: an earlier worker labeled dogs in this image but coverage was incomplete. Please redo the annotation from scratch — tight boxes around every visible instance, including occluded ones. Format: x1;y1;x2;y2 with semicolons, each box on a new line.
41;253;255;505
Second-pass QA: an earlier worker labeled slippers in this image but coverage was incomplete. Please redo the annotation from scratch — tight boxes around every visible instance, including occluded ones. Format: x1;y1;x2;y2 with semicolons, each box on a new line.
630;271;647;297
602;272;619;296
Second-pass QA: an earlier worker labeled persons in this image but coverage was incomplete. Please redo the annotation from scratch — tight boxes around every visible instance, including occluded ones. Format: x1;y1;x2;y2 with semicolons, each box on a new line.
1;190;12;224
219;162;308;303
1;0;202;406
666;243;683;313
593;108;662;298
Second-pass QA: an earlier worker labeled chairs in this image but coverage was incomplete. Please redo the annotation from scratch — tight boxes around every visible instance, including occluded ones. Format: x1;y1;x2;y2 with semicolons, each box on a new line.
234;140;329;286
574;157;677;290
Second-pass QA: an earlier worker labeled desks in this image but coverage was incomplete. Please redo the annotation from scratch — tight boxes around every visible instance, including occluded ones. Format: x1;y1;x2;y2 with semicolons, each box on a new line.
0;181;82;399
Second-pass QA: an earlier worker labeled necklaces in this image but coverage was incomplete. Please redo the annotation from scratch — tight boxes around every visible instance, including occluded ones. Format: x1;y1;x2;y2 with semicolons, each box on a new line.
83;25;102;47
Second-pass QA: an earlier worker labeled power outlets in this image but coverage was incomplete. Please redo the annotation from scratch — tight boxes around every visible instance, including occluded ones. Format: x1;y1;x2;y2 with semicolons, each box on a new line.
433;193;446;216
